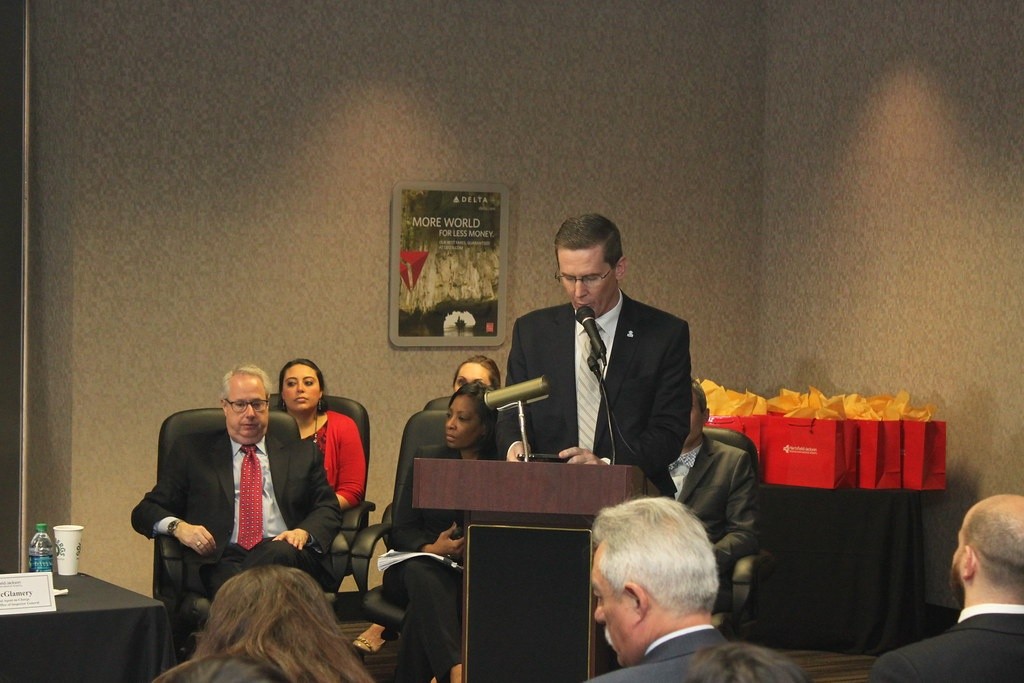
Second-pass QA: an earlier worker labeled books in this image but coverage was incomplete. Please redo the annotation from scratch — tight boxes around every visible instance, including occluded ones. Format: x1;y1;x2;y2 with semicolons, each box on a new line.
377;549;464;572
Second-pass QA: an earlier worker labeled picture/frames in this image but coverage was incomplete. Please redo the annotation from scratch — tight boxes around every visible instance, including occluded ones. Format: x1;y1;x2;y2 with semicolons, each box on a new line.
389;181;508;345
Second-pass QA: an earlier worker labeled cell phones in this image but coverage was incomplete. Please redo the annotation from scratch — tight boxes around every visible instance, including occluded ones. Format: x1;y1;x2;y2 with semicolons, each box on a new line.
518;453;571;463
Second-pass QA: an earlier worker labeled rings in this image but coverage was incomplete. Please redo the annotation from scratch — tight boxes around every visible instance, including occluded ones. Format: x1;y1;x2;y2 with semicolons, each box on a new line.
196;541;202;547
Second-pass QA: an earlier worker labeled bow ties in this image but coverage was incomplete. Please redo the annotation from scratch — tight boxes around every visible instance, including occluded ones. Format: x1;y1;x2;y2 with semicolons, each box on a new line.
668;449;696;471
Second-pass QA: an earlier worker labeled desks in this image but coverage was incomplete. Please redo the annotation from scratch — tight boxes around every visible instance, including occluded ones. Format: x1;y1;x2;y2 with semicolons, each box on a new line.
0;572;175;683
755;489;926;659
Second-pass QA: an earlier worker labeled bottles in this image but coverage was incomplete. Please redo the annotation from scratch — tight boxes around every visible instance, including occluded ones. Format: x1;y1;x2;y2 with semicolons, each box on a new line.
27;523;53;573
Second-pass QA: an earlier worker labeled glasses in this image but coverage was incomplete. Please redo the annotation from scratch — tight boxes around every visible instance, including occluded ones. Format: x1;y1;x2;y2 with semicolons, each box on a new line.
225;397;269;413
554;263;620;288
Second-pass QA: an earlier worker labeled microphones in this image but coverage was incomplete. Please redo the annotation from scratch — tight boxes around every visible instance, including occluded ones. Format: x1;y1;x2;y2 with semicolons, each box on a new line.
576;306;608;366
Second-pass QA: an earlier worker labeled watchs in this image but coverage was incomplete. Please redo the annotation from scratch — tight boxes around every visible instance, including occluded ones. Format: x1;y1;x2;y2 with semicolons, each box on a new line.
168;519;184;537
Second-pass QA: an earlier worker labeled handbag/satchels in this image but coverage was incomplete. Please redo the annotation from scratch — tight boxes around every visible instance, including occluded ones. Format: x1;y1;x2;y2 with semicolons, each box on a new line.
704;411;946;490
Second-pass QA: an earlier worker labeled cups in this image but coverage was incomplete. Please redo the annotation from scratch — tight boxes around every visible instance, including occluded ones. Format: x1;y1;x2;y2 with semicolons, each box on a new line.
52;524;84;576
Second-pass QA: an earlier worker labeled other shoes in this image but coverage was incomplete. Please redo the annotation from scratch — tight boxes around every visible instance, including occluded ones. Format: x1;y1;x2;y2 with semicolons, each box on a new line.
353;633;385;654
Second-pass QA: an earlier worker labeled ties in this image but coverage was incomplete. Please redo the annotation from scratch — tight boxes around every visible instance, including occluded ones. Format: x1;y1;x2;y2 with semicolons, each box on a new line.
237;445;263;551
575;332;602;454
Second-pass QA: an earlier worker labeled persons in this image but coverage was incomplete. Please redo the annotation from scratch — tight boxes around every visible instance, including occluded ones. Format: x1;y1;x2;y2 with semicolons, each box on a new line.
495;212;693;501
151;564;376;683
452;355;501;392
130;362;343;662
382;379;498;683
667;378;764;616
579;496;812;683
869;494;1024;683
278;358;366;510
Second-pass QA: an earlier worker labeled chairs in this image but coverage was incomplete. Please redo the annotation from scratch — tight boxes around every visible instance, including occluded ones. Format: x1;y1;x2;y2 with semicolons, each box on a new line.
268;394;376;536
695;429;775;636
153;406;300;645
381;396;453;549
348;411;451;630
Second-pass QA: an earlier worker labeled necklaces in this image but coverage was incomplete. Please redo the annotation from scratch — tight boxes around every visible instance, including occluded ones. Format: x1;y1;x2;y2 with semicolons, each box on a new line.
312;414;318;444
